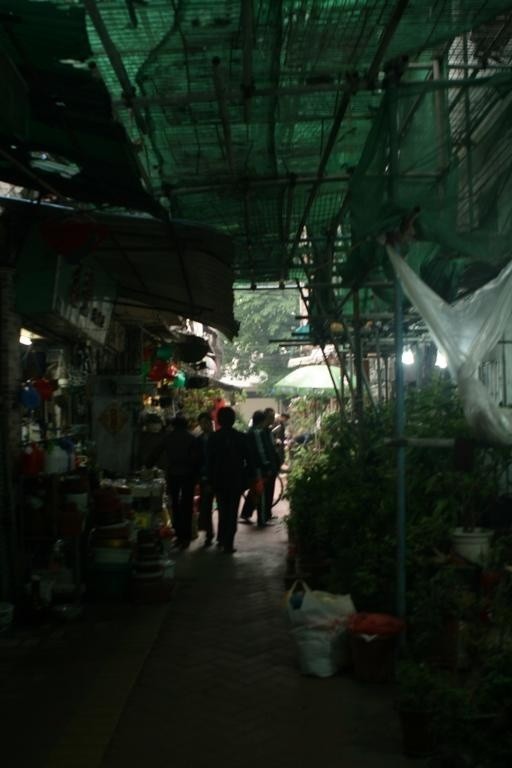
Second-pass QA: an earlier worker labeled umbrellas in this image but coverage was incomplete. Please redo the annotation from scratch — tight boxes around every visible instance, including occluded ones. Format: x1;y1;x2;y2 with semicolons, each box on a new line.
273;364;357;392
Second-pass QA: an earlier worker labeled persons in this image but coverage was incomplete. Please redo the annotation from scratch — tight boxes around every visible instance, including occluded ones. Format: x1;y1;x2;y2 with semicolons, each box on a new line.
139;406;316;554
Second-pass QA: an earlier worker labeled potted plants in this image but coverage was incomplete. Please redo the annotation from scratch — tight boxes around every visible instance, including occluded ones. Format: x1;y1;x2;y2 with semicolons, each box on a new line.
287;375;511;768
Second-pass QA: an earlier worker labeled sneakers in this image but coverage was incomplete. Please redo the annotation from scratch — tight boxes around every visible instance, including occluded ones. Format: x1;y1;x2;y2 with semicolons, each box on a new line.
205;533;237;553
240;513;279;526
